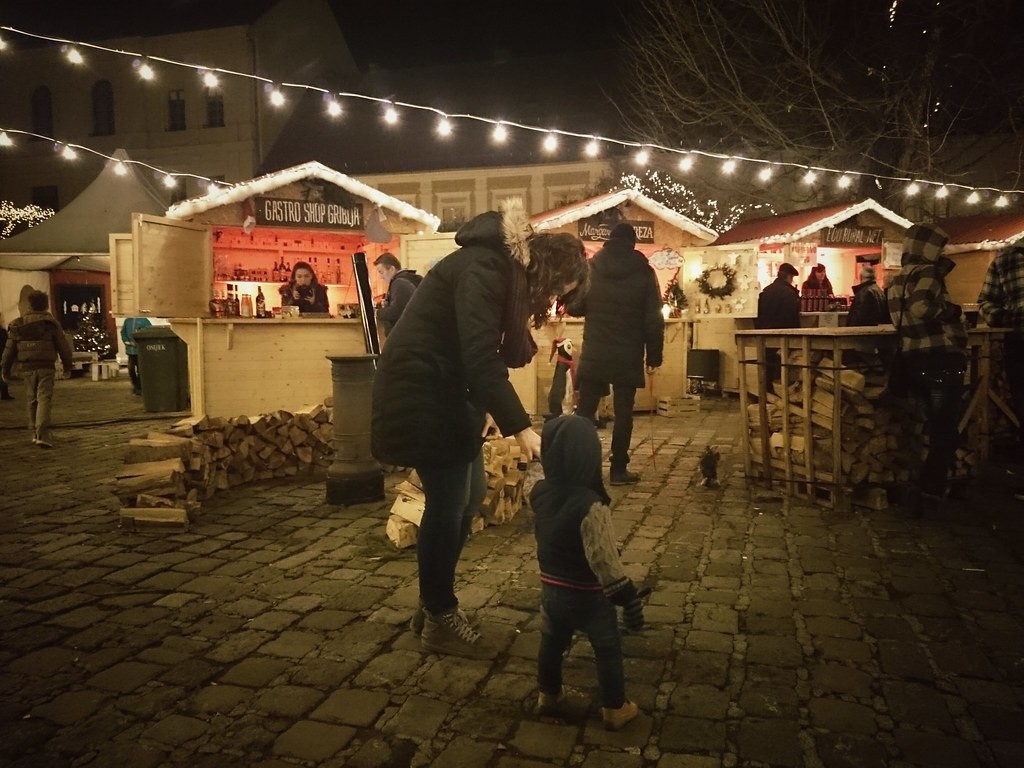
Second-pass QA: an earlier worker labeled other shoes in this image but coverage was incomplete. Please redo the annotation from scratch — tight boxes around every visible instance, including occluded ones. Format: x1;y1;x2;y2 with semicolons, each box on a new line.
32;439;37;442
2;396;15;400
134;386;142;395
36;440;52;448
1014;489;1024;500
610;470;639;485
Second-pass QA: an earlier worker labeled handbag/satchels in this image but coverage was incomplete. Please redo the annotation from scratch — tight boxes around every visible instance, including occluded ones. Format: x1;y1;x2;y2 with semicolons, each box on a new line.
889;354;909;399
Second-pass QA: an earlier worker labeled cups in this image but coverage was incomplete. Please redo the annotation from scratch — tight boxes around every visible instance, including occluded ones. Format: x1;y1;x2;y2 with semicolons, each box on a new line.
962;304;980;330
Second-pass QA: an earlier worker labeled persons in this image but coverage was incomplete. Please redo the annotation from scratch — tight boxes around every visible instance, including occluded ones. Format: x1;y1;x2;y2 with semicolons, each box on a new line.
802;263;834;299
0;291;73;449
278;261;330;314
565;223;666;487
522;415;644;731
120;318;152;397
752;263;801;393
373;252;423;338
0;311;16;400
885;222;981;493
977;239;1024;501
370;199;589;660
845;266;893;326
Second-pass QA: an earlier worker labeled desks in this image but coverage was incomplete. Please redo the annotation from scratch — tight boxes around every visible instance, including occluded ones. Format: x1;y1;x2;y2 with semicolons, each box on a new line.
734;326;1014;514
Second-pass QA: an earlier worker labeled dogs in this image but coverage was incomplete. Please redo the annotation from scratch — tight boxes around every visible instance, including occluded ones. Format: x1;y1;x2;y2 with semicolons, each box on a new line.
697;444;722;489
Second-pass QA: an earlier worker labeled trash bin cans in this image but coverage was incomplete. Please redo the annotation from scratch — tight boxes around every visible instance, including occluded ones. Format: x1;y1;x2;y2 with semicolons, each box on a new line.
134;326;190;412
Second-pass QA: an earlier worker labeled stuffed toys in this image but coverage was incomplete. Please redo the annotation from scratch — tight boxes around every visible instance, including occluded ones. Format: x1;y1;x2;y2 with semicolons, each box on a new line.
548;337;577;417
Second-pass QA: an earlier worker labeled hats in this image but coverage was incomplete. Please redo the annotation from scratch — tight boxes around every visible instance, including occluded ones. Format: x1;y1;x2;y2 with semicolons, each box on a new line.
779;263;798;276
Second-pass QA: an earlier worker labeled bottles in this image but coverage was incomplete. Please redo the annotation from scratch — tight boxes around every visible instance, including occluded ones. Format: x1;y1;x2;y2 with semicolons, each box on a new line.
216;257;342;284
209;284;300;319
694;298;743;313
799;289;856;312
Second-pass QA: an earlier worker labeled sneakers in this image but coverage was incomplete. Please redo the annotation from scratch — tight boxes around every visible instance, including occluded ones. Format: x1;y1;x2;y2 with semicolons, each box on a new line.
538;684;592;711
601;702;638;731
421;607;498;659
410;596;481;638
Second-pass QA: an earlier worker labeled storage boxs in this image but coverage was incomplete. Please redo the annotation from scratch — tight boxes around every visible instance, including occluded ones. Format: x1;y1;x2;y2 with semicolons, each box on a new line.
656;394;701;418
